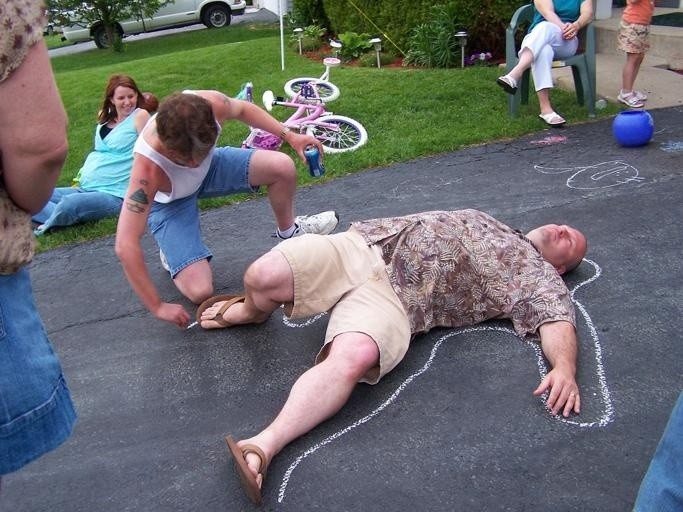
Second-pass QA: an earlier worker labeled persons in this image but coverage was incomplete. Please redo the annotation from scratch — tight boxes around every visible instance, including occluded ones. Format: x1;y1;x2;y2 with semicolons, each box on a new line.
0;1;76;472
114;89;325;330
196;206;588;505
618;0;655;107
497;0;595;127
629;391;683;511
31;76;154;239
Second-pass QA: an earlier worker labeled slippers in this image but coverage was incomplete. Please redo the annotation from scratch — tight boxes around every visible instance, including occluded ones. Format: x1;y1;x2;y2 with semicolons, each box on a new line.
196;295;245;329
497;75;518;95
224;434;267;503
538;111;567;126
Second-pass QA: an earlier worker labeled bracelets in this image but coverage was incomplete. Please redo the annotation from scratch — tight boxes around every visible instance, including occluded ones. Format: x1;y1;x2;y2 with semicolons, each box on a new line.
280;128;290;140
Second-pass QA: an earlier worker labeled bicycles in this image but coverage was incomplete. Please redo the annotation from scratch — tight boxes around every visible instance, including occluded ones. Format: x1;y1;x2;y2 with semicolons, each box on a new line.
243;58;368;167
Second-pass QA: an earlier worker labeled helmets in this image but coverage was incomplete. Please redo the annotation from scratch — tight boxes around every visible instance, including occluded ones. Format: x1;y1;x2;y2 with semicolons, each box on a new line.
141;93;159;112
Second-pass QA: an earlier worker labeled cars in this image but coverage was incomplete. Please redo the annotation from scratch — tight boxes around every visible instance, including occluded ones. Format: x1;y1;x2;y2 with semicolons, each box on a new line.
62;0;247;50
41;4;93;35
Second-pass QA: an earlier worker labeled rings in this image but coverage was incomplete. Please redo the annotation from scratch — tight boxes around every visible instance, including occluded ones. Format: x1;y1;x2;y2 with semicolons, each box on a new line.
569;393;575;397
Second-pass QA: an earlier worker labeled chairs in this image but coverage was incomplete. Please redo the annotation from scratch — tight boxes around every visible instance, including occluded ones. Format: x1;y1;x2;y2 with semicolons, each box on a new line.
505;4;596;117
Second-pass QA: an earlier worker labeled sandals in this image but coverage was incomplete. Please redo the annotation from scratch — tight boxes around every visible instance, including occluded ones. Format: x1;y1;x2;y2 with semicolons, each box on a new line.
617;90;648;108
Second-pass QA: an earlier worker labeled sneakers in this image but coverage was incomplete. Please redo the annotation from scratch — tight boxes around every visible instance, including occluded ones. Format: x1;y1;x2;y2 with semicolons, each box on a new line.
275;211;339;244
